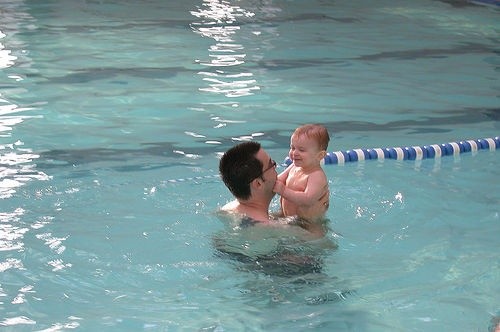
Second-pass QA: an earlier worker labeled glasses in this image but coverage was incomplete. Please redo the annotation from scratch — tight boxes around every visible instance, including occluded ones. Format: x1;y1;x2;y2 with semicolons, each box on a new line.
247;159;276;184
